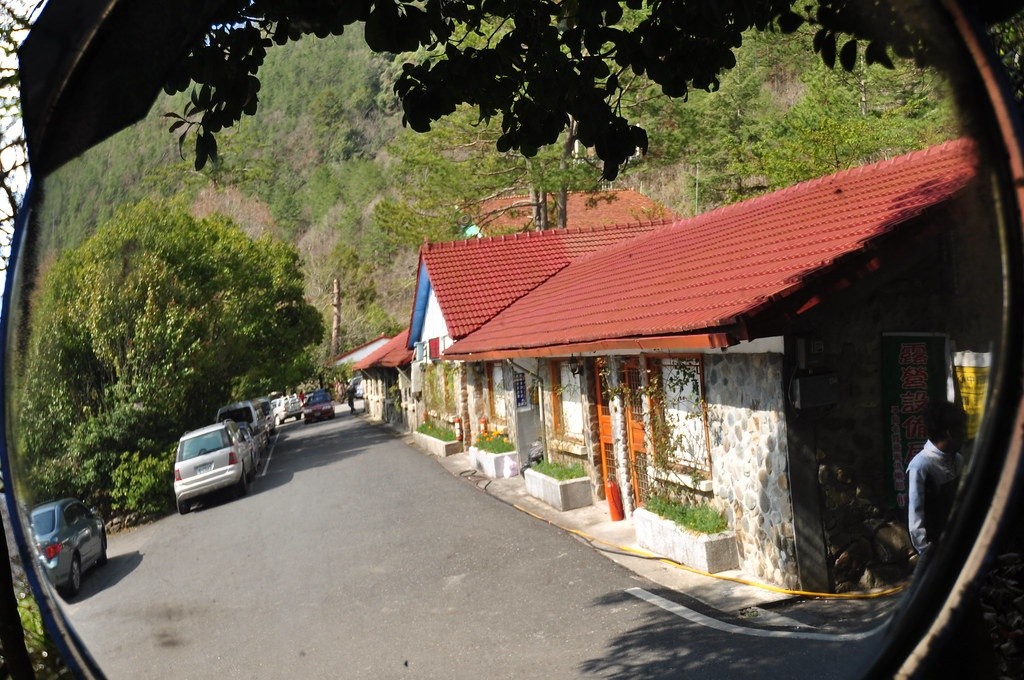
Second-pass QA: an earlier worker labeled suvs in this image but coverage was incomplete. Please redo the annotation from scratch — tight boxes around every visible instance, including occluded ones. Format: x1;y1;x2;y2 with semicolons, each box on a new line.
272;397;303;425
173;418;257;516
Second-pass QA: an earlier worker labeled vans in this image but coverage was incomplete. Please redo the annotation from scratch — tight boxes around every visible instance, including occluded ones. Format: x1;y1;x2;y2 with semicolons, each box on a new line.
253;396;277;436
216;400;271;452
350;377;362;392
356;381;364;398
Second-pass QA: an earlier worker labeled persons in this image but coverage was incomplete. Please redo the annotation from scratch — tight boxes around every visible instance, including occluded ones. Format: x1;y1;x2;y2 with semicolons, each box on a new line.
905;402;969;556
299;392;304;404
344;382;356;414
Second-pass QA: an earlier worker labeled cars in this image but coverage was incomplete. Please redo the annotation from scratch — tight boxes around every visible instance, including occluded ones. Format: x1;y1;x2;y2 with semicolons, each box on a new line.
198;421;261;473
31;499;108;598
303;392;335;424
